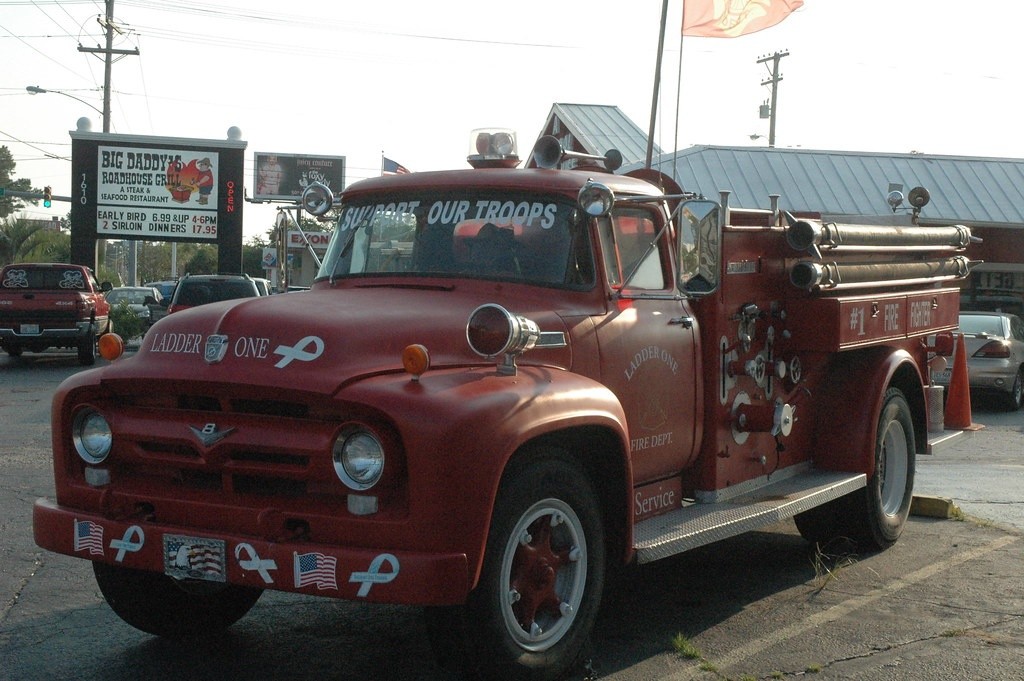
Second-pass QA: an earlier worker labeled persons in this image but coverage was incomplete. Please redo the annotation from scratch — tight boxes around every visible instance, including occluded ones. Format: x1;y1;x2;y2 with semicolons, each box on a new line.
258;155;280;194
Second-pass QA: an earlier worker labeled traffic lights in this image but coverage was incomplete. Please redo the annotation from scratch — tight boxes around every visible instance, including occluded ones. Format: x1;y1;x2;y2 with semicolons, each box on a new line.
43;186;53;208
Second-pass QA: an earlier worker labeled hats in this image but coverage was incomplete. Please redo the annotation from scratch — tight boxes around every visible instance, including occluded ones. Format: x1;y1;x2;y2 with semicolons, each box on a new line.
477;222;502;243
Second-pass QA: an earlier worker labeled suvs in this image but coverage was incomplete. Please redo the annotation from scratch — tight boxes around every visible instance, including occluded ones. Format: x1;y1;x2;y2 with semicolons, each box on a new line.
160;273;262;317
0;264;115;367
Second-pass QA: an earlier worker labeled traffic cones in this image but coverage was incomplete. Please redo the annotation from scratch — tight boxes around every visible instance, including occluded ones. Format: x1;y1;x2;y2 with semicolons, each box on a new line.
942;332;985;431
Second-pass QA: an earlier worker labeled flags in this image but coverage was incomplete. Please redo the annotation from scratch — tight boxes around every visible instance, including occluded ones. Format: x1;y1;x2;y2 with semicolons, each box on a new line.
382;157;410;176
681;0;803;38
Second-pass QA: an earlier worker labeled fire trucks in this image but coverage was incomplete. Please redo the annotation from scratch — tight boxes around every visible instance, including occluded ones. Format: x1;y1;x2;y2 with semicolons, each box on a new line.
32;130;986;681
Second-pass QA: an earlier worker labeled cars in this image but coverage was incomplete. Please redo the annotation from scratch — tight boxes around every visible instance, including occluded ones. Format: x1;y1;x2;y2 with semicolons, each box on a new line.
142;279;177;327
926;311;1024;410
253;276;273;298
103;287;161;339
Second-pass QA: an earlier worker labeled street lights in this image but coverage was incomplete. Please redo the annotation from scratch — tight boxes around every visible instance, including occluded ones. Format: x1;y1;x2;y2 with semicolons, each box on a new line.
23;84;112;135
